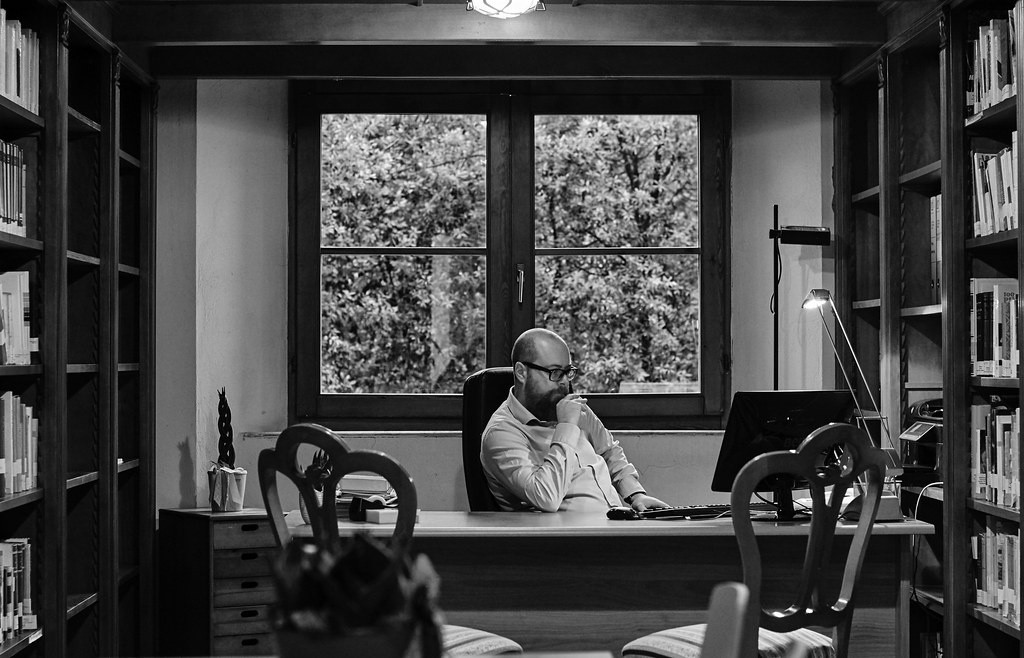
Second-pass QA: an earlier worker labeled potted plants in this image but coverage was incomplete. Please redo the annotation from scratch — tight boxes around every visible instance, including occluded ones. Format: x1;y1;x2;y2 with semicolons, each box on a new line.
293;452;345;526
207;385;248;511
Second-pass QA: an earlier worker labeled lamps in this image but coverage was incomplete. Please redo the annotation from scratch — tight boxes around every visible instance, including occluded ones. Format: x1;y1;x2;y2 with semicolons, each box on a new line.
466;0;546;19
801;289;904;520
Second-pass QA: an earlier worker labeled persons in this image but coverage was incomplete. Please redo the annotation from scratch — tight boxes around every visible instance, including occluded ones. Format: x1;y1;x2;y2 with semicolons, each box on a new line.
479;327;673;514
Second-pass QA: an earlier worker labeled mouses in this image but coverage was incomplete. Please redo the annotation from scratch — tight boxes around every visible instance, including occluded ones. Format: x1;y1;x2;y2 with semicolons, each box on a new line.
606;506;636;520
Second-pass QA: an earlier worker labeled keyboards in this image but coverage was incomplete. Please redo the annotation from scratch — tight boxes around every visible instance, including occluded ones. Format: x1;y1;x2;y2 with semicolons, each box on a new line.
639;503;779;518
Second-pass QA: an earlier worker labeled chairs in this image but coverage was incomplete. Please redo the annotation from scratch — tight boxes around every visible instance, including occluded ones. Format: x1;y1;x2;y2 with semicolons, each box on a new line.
622;424;887;658
257;422;523;658
462;367;531;512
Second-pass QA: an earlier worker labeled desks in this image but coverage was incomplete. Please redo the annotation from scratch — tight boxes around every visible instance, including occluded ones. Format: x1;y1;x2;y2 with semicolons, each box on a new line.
284;510;936;658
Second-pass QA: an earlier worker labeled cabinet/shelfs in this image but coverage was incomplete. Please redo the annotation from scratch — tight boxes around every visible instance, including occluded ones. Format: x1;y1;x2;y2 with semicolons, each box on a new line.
159;507;291;658
951;0;1023;658
0;0;161;658
830;0;951;658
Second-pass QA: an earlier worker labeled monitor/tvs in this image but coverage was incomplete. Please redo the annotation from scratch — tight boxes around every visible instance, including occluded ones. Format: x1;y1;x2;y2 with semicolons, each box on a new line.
711;388;858;522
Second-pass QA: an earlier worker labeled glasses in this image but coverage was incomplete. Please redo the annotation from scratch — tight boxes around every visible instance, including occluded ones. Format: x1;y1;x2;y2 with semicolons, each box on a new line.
521;362;579;382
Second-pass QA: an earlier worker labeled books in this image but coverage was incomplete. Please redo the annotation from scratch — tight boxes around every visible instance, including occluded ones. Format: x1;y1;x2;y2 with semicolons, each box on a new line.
926;0;1023;635
0;9;40;644
